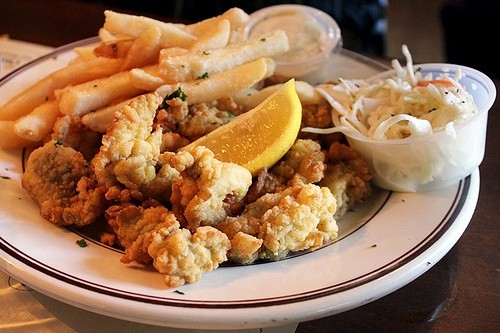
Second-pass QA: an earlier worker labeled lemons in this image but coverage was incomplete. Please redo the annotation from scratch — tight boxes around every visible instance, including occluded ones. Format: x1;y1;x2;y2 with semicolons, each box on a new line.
176;77;303;177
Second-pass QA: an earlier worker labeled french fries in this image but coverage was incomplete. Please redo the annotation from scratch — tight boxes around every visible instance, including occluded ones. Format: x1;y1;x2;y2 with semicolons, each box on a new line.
1;7;289;150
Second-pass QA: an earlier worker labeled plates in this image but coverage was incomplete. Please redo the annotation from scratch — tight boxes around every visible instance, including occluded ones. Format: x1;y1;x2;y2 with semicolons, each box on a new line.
0;36;480;330
